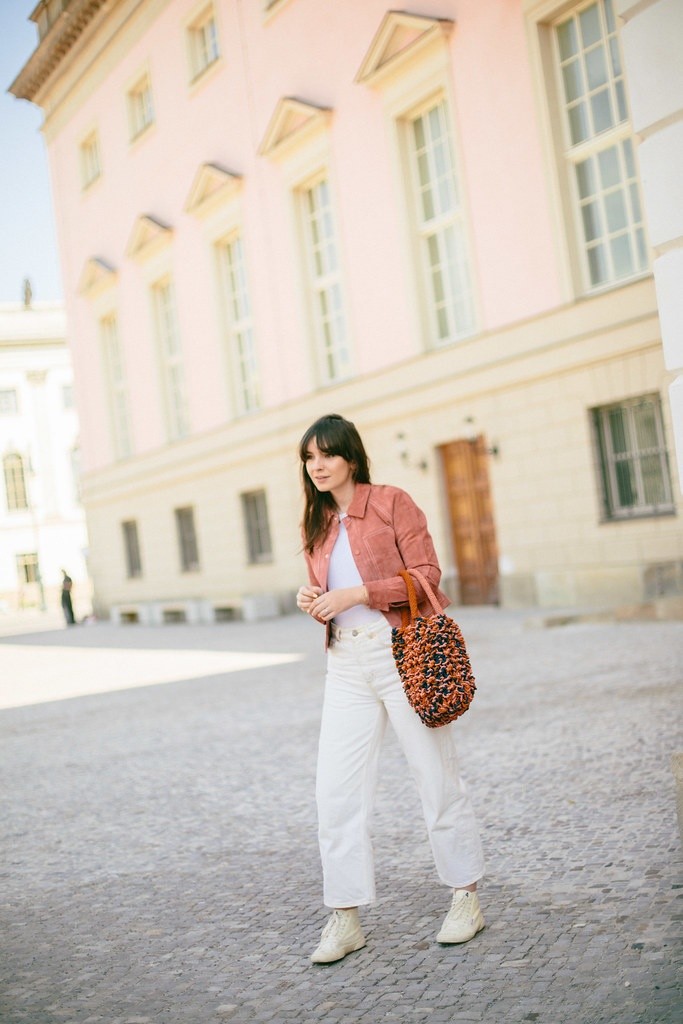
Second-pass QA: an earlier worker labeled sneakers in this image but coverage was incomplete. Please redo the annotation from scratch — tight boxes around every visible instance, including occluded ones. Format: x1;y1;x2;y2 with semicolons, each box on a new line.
310;907;366;963
436;888;485;944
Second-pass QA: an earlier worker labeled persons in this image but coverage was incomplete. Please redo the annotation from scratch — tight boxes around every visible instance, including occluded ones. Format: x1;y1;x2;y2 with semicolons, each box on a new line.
61;570;77;624
296;414;486;965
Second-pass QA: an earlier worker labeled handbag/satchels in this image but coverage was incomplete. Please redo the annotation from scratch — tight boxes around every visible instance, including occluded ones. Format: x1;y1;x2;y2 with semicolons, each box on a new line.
391;568;475;730
